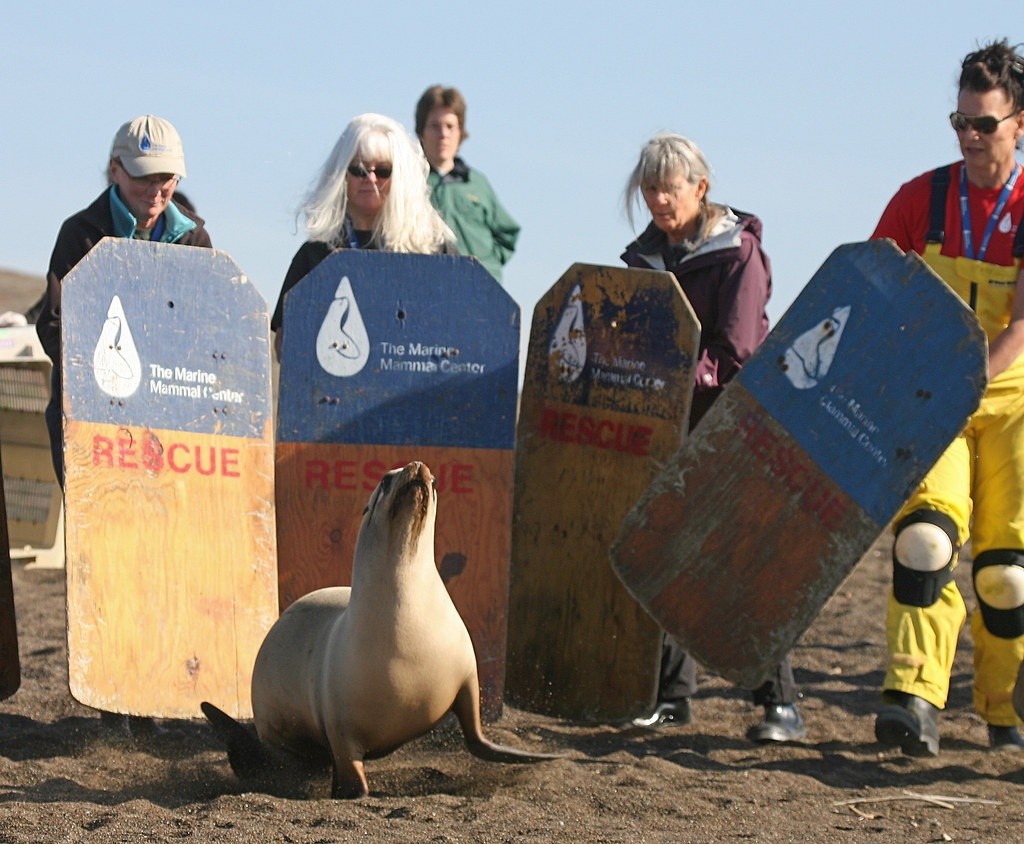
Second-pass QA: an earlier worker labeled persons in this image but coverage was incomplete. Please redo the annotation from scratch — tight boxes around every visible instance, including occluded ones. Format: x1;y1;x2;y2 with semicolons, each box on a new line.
614;133;813;747
25;115;213;487
870;37;1024;759
408;82;524;289
256;109;467;348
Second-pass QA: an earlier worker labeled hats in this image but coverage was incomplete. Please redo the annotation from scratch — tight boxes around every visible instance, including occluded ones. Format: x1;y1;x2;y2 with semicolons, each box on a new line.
110;115;187;177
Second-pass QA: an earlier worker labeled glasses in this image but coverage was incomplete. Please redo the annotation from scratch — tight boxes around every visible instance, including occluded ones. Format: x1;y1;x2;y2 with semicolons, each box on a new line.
346;164;391;181
950;110;1018;134
113;160;179;183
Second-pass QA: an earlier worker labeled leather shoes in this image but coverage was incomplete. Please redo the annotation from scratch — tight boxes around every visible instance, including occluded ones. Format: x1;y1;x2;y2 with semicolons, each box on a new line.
747;705;808;743
631;698;690;730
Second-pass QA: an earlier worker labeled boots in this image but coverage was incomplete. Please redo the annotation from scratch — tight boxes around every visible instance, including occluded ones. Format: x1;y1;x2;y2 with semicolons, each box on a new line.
988;724;1024;753
874;694;940;757
